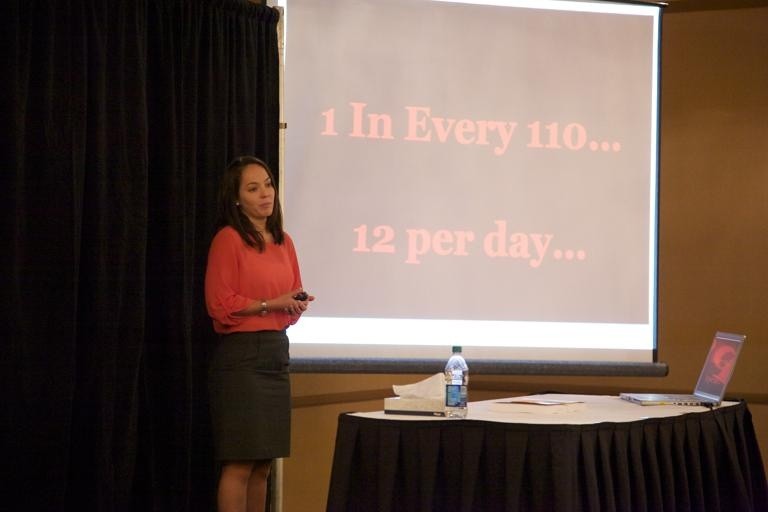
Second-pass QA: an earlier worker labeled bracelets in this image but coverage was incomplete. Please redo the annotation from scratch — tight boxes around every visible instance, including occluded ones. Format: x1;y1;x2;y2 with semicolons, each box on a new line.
260;299;267;317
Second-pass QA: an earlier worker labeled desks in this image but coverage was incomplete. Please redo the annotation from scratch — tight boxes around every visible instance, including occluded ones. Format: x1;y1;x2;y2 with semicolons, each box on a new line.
325;395;759;512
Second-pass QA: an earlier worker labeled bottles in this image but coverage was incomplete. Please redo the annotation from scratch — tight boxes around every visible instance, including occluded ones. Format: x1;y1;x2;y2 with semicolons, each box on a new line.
445;346;469;417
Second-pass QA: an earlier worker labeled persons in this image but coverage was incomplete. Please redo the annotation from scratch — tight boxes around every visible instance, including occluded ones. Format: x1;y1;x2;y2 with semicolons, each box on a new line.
204;156;314;512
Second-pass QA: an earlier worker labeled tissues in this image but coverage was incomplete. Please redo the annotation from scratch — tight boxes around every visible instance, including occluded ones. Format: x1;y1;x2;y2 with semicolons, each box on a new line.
383;368;446;416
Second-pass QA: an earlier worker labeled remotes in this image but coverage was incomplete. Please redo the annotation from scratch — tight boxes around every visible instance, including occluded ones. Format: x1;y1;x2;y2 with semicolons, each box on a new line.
295;292;308;300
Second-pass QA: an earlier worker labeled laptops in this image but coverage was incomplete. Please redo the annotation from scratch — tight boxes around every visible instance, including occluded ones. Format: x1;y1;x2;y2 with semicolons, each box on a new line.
619;331;747;407
